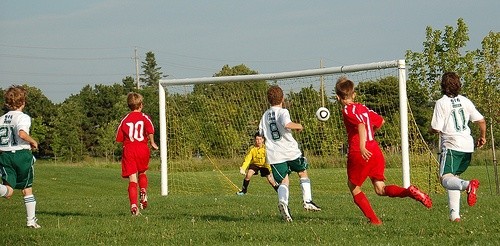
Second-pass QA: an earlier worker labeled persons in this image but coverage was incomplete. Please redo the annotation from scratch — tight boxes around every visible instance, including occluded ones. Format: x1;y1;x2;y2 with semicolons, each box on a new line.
336;76;431;226
115;92;159;215
236;132;280;196
0;87;42;229
429;72;486;223
258;85;322;221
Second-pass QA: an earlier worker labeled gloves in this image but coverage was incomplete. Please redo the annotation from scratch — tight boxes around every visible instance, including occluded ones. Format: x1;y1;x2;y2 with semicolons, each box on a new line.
239;167;246;175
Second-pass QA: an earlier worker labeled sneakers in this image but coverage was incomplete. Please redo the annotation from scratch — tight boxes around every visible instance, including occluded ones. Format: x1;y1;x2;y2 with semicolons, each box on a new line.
466;180;480;207
139;188;148;210
302;200;321;212
26;217;41;229
449;218;461;222
236;191;247;196
367;220;382;224
407;185;433;208
278;202;294;222
130;203;138;215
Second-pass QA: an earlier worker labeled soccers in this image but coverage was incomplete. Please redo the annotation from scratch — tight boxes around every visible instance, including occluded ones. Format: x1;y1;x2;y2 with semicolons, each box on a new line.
316;107;330;121
